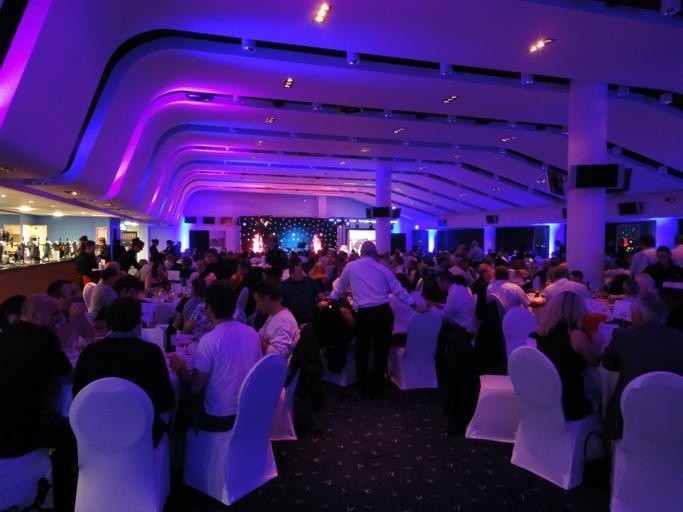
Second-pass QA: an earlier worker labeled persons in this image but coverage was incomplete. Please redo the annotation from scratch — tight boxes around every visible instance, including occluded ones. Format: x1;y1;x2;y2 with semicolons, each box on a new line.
482;234;682;489
0;232;324;487
308;239;538;389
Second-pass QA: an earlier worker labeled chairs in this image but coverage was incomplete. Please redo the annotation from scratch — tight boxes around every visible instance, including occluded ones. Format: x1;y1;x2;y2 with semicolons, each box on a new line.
505;345;593;492
2;258;681;389
610;371;680;512
181;353;285;505
270;321;309;441
464;373;517;445
69;378;169;510
1;444;52;510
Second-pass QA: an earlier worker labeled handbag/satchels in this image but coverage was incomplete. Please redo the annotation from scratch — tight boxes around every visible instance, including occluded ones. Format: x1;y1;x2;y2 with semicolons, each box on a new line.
582;431;611;489
